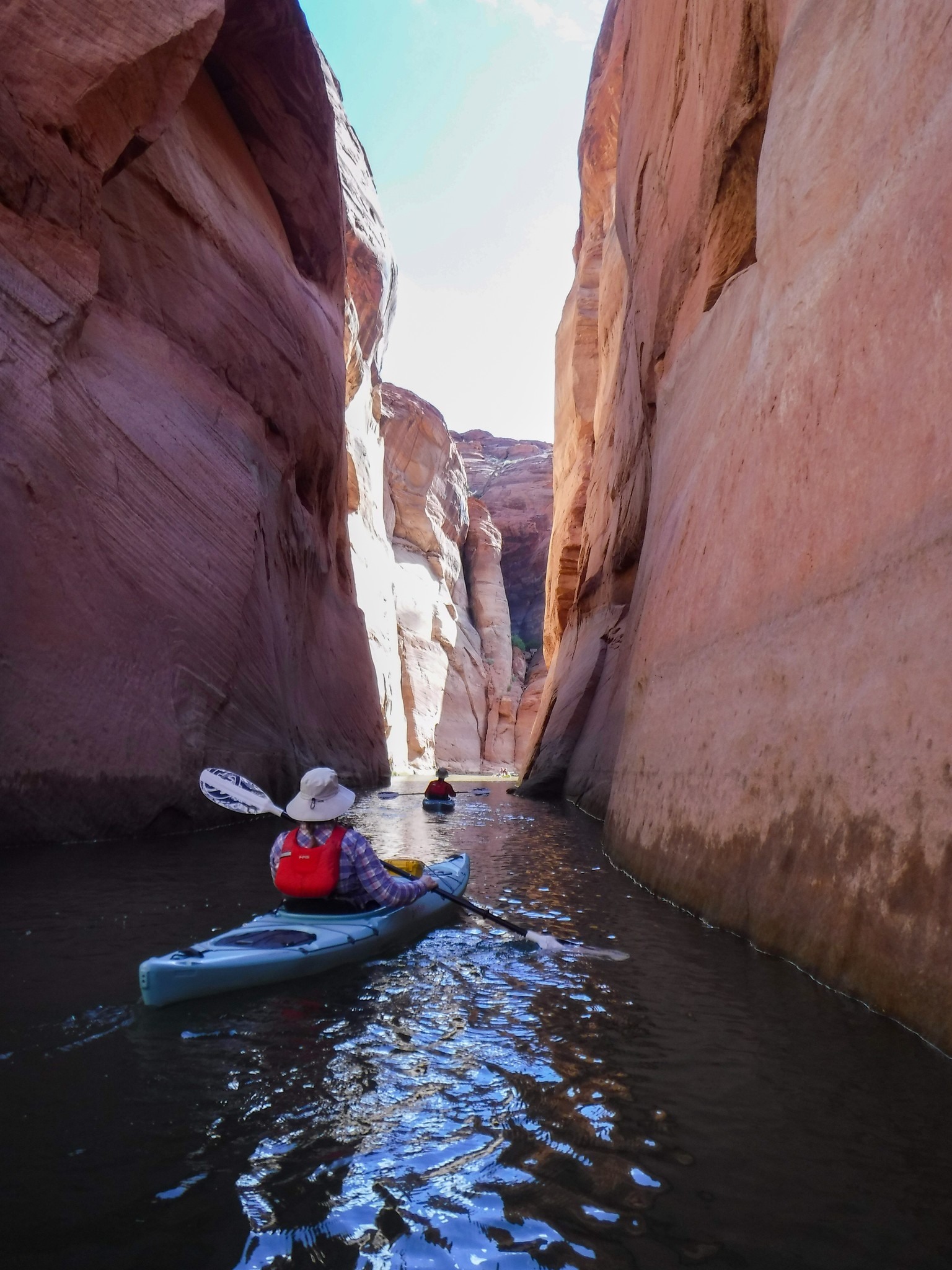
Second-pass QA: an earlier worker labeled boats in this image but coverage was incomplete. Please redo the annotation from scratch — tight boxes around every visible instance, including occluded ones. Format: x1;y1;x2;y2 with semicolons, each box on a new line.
422;795;455;813
136;852;470;1010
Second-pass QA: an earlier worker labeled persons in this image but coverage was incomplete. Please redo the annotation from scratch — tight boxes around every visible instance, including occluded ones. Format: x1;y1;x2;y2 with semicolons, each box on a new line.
499;768;508;774
424;767;456;800
270;768;439;908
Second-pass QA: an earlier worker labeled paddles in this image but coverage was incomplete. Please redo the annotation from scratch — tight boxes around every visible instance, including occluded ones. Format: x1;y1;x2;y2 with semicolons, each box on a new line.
377;787;490;801
197;762;630;962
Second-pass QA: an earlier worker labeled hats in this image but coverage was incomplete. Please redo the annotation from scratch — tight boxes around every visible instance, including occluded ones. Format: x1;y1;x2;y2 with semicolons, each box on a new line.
286;768;356;822
436;768;448;778
